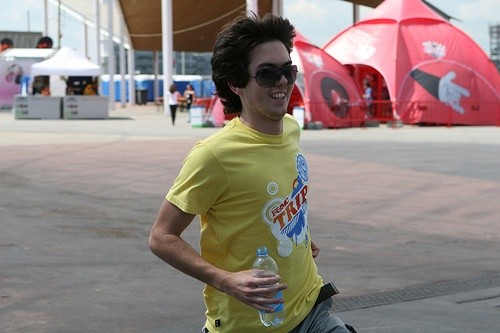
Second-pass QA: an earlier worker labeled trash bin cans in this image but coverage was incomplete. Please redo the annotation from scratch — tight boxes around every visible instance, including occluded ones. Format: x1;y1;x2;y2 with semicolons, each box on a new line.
136;87;148;104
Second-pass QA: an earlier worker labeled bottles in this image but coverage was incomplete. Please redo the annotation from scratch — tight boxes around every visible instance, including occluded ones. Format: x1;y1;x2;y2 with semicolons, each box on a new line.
253;248;284;327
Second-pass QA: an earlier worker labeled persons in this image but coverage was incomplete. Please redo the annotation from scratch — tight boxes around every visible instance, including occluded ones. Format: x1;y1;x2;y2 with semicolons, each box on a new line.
183;83;195;122
149;11;357;333
169;84;181;125
41;83;98;96
361;80;373;118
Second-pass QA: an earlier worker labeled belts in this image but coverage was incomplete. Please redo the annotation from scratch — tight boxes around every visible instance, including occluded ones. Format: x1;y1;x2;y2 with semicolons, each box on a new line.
313;281;340;305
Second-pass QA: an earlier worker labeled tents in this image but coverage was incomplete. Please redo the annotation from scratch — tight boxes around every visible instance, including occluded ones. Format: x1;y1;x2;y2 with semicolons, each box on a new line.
203;31;363;130
320;0;500;127
31;47;101;76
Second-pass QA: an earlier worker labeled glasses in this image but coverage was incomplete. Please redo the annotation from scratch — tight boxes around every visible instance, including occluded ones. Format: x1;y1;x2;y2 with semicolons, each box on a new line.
246;65;298;87
